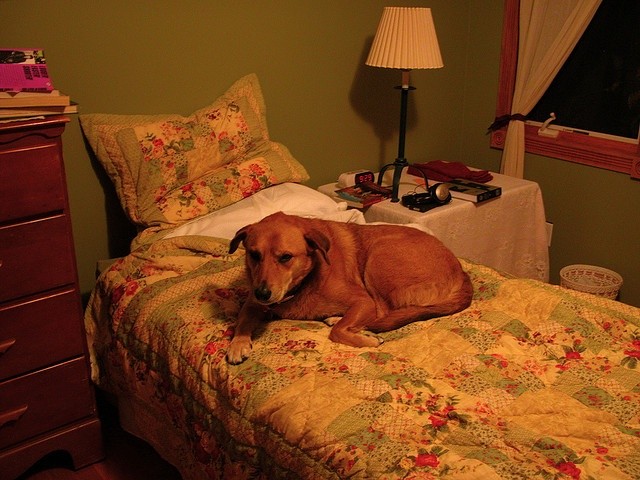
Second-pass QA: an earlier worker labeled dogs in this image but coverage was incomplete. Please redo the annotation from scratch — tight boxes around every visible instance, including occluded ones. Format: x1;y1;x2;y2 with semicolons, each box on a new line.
225;211;473;366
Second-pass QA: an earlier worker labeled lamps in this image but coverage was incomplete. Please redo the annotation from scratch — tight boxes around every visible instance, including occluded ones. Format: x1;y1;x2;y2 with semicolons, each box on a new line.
364;6;438;203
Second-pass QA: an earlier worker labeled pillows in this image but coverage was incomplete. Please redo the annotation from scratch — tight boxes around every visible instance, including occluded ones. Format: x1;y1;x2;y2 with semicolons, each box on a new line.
78;72;310;254
167;179;340;240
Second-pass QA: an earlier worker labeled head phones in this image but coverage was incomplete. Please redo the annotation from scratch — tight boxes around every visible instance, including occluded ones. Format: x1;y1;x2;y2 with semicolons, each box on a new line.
402;183;451;212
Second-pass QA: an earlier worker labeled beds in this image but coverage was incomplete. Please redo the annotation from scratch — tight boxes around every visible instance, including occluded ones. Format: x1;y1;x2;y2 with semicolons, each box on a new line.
96;224;640;480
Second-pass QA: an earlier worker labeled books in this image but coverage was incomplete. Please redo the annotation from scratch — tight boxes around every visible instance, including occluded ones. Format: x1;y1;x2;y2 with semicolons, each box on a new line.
334;180;391;208
444;178;502;203
0;48;78;119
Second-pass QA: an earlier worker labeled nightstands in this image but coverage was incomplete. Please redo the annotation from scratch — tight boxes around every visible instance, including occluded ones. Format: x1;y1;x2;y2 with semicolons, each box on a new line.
319;157;538;276
2;114;106;476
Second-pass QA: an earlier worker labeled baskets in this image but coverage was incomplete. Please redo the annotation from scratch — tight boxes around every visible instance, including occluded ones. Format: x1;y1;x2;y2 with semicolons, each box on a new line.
559;264;624;300
